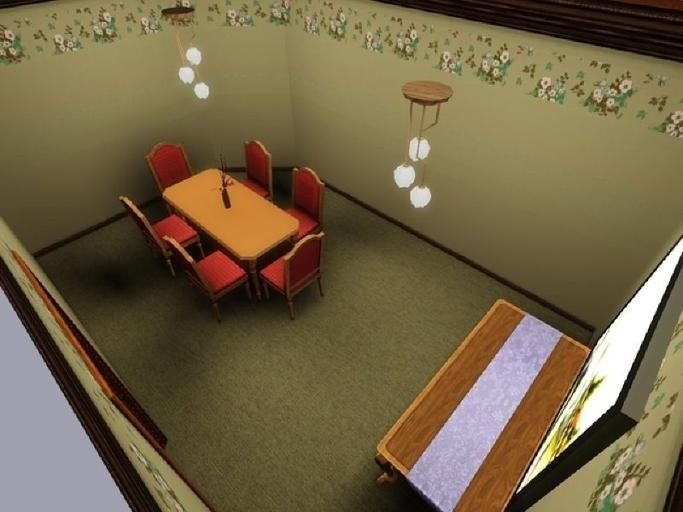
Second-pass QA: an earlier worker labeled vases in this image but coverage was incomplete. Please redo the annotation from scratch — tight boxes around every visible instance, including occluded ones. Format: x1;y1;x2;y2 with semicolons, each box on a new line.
222;189;231;209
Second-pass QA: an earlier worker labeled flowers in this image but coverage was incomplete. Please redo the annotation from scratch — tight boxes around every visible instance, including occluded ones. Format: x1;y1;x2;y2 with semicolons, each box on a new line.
214;153;235;191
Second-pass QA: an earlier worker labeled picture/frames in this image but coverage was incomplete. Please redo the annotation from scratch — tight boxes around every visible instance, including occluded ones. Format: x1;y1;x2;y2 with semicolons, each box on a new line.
6;246;169;453
505;230;683;512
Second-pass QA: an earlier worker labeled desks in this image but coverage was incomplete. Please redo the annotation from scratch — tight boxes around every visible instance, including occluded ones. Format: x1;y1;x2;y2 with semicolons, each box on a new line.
373;299;591;512
162;167;299;302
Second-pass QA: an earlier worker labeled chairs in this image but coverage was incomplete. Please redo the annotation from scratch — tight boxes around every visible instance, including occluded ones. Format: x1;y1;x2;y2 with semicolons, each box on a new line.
232;140;274;205
118;194;205;278
161;234;253;324
146;140;194;232
259;232;327;319
280;167;325;245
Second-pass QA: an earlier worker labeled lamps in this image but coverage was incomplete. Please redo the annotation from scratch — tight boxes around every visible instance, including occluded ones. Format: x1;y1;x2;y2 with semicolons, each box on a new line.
388;81;453;209
160;6;210;99
